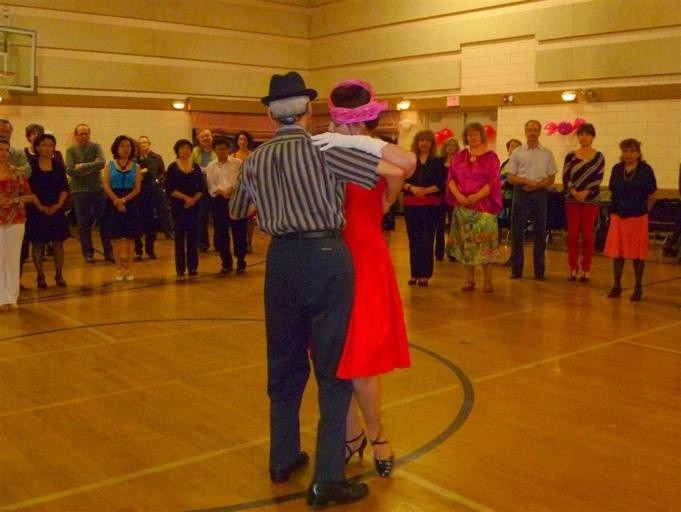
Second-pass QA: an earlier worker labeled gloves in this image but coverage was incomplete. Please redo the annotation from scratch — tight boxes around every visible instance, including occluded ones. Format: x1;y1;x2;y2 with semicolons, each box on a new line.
310;131;388;158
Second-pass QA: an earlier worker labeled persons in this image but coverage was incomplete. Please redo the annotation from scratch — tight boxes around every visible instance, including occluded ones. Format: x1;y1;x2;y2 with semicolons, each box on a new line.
434;136;461;262
561;123;605;284
207;135;251;274
309;77;419;477
491;139;524;268
605;138;658;302
189;127;222;256
400;128;445;288
444;122;502;293
506;119;559;281
0;119;166;312
166;138;207;276
226;71;405;507
228;130;258;254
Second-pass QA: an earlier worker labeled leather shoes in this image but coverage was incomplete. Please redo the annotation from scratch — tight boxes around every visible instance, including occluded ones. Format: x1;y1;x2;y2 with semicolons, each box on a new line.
54;273;65;287
269;450;308;484
125;271;134;280
306;481;367;508
115;271;123;281
37;274;46;289
407;272;642;302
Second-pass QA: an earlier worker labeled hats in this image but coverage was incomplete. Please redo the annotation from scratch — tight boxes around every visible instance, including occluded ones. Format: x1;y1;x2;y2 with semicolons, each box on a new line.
260;71;317;107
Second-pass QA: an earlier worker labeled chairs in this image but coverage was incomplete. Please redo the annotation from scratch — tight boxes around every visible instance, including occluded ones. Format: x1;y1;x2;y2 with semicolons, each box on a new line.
647;199;680;262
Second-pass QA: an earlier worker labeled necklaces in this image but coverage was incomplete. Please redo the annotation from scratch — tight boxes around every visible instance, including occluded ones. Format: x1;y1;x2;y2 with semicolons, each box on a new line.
623;161;638;180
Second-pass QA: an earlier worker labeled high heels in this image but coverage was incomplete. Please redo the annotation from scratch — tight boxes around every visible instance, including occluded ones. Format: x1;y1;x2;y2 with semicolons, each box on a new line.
369;425;392;478
345;432;366;464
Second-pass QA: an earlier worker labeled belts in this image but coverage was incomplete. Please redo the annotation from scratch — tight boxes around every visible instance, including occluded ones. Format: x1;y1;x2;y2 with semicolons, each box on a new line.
277;230;341;240
